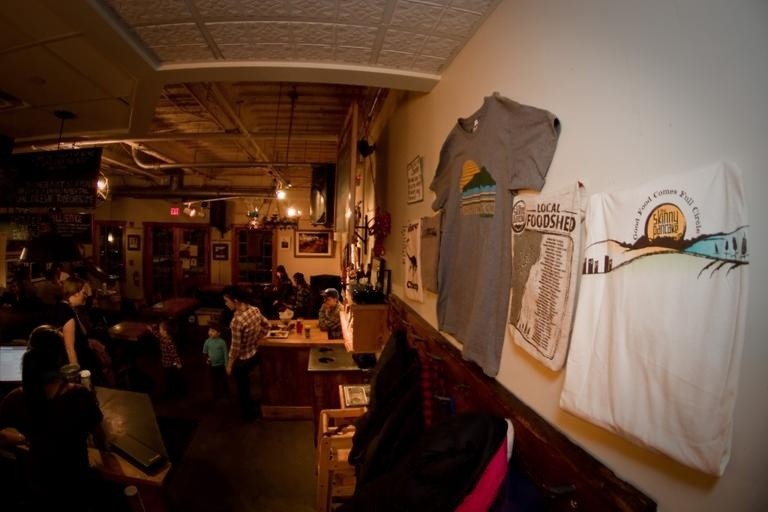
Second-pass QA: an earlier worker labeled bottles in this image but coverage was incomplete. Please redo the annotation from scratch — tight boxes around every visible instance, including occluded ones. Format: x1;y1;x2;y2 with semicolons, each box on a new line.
296;320;310;338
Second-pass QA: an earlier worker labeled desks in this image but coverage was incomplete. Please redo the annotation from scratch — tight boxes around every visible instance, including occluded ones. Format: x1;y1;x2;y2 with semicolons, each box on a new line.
308;345;373;449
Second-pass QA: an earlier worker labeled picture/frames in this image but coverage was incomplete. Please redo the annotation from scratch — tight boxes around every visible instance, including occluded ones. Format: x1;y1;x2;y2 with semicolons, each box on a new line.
127;235;140;250
294;228;334;258
212;244;229;260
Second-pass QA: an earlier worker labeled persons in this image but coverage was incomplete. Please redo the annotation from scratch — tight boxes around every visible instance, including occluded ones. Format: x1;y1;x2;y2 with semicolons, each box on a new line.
220;283;271;422
202;323;232;404
245;261;317;320
318;286;345;340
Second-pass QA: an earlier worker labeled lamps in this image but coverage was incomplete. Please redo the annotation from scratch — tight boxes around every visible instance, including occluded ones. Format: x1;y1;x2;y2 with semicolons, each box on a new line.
182;196;239;217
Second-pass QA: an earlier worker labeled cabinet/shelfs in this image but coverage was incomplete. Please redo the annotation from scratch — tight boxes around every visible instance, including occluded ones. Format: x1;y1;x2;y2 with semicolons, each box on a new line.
347;302;388;354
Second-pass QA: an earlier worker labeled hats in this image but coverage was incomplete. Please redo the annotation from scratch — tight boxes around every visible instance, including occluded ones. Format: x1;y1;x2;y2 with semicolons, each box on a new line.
321;288;340;298
